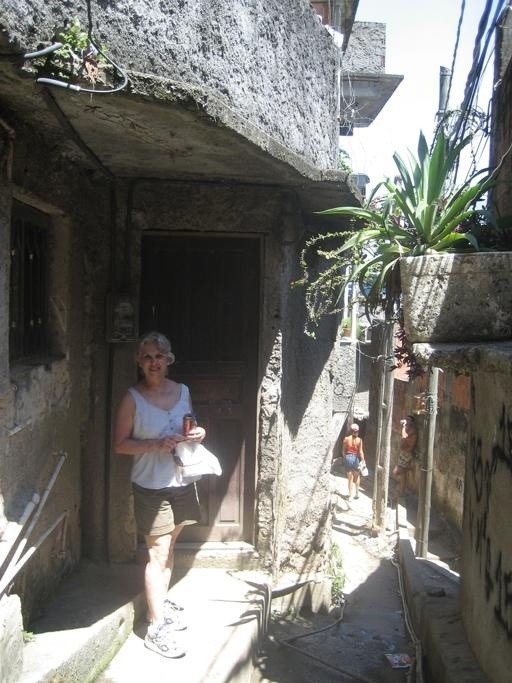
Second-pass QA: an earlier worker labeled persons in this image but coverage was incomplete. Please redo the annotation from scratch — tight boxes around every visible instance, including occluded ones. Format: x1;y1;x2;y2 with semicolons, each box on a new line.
111;329;208;658
342;423;366;502
391;414;417;497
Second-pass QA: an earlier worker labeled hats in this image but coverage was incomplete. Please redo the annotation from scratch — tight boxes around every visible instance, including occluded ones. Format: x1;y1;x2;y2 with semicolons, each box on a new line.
171;439;223;479
350;423;359;430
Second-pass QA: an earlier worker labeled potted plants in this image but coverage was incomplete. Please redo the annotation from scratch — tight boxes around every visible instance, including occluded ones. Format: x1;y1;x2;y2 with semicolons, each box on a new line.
288;108;511;343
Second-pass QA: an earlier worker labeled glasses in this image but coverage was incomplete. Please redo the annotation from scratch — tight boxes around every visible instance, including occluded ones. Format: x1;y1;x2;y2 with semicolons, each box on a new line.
170;437;201;468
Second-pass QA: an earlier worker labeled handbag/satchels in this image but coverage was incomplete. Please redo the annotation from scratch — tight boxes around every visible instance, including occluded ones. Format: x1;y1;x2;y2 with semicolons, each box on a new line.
357;458;368;477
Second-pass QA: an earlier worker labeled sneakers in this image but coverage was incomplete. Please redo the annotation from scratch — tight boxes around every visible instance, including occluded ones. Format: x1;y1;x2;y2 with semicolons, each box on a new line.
143;623;188;658
144;608;189;630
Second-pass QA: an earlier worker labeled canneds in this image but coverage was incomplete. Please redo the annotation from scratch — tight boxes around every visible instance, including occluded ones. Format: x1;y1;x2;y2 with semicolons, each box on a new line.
183;414;194;436
